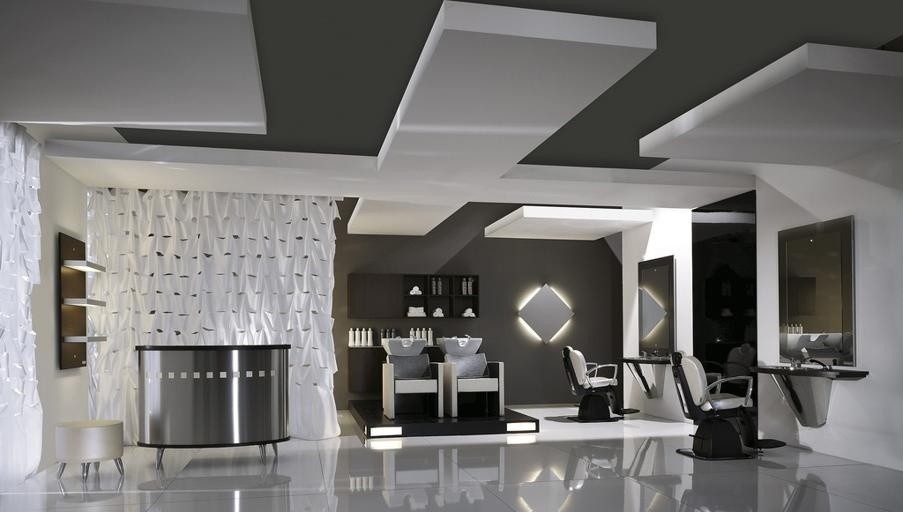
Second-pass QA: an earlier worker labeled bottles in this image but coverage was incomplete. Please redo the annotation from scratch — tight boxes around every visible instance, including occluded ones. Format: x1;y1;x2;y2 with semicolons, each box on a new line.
461;277;467;295
787;323;803;335
391;328;396;338
348;327;355;348
427;327;433;347
367;327;374;347
380;328;385;338
438;277;442;295
421;327;427;347
354;327;361;348
361;327;367;347
409;327;415;339
415;327;421;339
431;278;437;295
386;328;391;338
467;277;473;295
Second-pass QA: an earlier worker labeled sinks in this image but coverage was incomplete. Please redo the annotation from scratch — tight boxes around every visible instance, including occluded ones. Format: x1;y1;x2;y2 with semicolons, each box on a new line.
616;357;670;399
382;487;429;508
381;338;427;357
780;332;843;356
781;472;831;512
436;337;482;355
748;366;869;428
444;482;485;505
613;436;682;500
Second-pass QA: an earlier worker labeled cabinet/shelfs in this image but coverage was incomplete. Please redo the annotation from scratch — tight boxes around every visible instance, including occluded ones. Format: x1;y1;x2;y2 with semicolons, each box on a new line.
347;273;480;320
57;231;106;370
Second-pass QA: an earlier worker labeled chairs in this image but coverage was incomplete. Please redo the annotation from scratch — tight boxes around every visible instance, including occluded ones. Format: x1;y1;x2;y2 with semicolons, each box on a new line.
668;350;785;459
561;345;640;423
381;352;505;420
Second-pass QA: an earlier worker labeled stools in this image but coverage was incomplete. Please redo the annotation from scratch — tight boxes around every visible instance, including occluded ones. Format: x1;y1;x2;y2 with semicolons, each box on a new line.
55;419;125;481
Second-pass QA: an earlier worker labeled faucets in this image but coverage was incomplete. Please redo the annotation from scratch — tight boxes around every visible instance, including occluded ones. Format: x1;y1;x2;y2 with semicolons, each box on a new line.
809;359;827;368
651;352;660;357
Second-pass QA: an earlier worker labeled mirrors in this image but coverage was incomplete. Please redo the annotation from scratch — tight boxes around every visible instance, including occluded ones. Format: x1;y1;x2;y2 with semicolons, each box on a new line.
638;254;674;357
778;215;855;367
